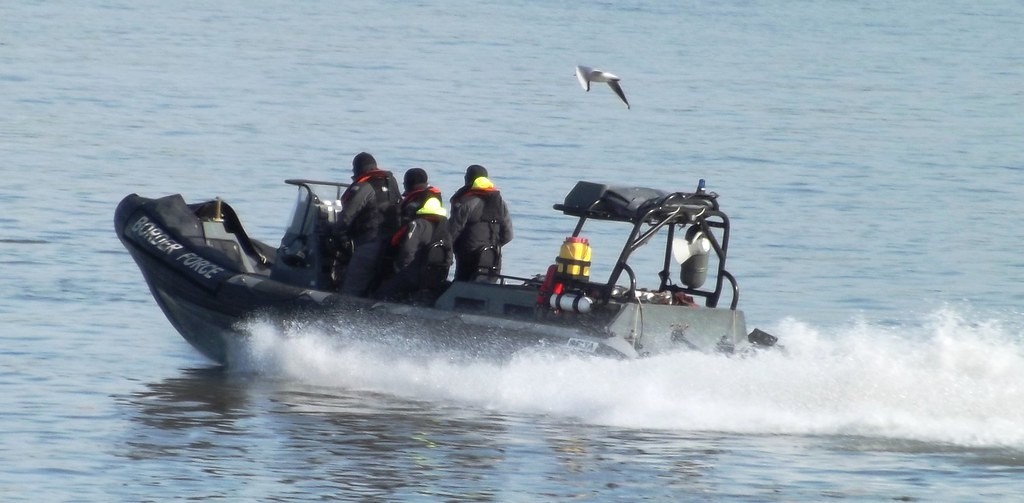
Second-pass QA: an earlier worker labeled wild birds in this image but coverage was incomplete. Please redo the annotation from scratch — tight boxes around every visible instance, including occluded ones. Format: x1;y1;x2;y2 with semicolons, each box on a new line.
573;65;630;111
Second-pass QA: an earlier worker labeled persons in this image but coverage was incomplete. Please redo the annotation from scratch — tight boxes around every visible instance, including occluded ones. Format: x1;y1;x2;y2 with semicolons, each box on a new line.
334;152;513;304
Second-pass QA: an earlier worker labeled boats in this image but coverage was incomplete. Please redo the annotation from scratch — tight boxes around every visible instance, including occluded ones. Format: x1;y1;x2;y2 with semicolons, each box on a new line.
114;176;786;375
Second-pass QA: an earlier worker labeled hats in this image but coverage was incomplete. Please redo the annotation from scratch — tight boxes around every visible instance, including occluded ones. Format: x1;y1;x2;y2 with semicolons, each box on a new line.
353;152;377;177
465;165;487;187
420;186;442;208
404;168;428;191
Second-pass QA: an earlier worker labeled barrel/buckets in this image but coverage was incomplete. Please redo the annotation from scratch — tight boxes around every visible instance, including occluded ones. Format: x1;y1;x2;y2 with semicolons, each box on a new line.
557;237;591;281
